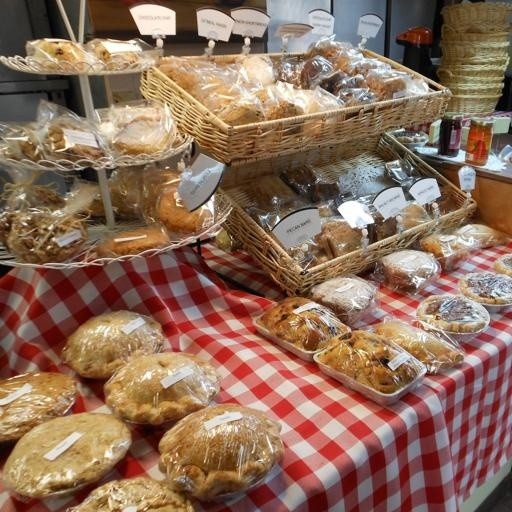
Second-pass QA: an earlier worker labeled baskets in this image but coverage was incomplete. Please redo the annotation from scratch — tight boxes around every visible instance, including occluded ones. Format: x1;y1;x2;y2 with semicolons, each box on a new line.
437;0;512;118
214;133;478;298
139;47;452;168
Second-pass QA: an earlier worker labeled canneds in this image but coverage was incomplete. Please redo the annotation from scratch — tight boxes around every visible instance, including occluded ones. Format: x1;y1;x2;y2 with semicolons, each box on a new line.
466;119;493;165
438;116;462;156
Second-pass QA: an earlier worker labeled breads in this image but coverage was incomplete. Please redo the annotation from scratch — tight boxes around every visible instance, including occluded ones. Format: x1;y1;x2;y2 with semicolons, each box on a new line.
494;254;512;275
373;249;442;293
65;478;195;512
454;223;500;250
308;182;342;201
157;403;286;503
0;166;213;263
420;236;470;273
0;371;79;444
458;271;512;304
316;189;360;218
0;412;133;502
280;164;319;193
310;274;380;324
256;295;350;350
250;174;294;206
366;215;395;245
397;201;433;231
319;328;427;394
25;39;141;72
416;294;490;332
0;106;170;166
61;310;164;379
103;352;221;425
307;222;362;265
160;38;409;125
367;316;465;366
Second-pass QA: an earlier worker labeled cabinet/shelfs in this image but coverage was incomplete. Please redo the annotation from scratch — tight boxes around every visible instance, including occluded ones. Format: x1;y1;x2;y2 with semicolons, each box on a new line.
409;144;512;239
0;221;512;512
